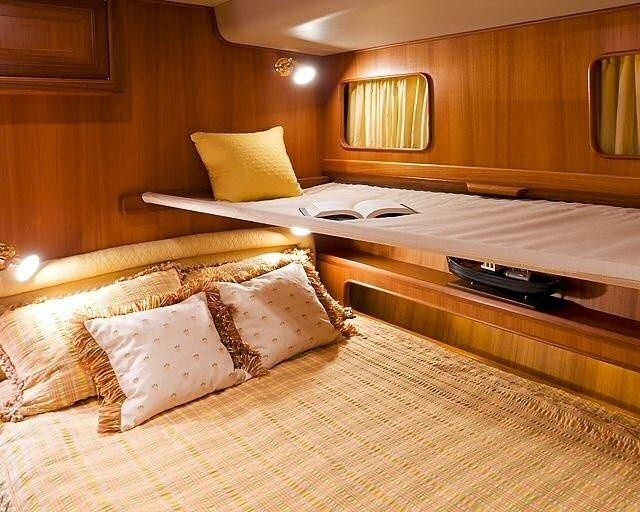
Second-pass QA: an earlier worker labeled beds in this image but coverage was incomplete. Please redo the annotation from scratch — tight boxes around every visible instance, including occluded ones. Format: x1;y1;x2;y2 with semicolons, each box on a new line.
0;226;639;512
120;177;639;288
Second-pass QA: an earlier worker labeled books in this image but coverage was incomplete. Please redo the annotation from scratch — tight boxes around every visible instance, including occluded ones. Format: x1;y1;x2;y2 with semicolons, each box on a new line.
298;198;418;221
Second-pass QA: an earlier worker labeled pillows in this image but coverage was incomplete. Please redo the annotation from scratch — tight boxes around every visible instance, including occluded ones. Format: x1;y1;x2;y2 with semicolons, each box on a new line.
0;259;188;423
62;274;271;432
184;246;359;378
190;125;303;202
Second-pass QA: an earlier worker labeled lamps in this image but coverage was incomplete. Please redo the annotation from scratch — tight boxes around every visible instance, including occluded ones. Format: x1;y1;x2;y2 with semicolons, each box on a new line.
275;58;315;85
